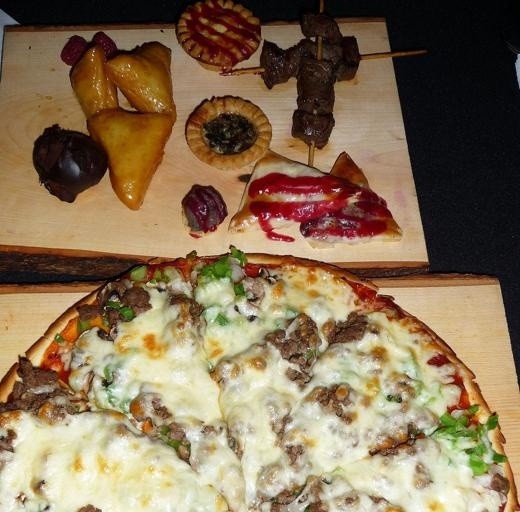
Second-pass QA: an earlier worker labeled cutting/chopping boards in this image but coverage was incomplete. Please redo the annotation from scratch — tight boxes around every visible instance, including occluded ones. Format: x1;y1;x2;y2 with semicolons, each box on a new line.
0;14;431;278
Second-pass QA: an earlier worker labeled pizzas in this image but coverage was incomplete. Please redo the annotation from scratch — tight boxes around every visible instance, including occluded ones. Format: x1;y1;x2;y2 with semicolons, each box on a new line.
228;147;380;234
300;151;402;247
